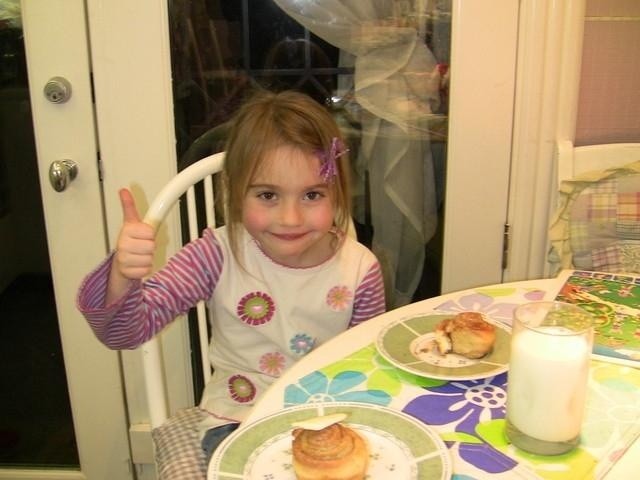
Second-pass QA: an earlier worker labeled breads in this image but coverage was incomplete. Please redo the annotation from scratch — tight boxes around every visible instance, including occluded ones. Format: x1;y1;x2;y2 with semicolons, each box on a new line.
291;413;371;480
436;312;497;359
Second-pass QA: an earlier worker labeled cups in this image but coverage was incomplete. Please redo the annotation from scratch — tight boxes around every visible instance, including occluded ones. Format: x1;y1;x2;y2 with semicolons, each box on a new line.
502;299;596;457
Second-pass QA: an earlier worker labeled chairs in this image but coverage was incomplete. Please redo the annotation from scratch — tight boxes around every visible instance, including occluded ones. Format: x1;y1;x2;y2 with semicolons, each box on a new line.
140;144;368;480
558;131;639;276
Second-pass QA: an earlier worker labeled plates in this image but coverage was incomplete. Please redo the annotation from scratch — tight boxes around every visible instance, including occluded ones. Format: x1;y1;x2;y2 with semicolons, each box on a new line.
373;309;513;382
206;399;453;480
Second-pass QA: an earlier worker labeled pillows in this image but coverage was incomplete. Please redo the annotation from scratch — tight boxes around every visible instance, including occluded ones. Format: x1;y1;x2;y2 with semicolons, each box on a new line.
549;160;639;275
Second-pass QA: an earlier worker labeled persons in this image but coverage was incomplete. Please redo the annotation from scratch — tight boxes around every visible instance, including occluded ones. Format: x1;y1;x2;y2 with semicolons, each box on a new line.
74;91;388;465
